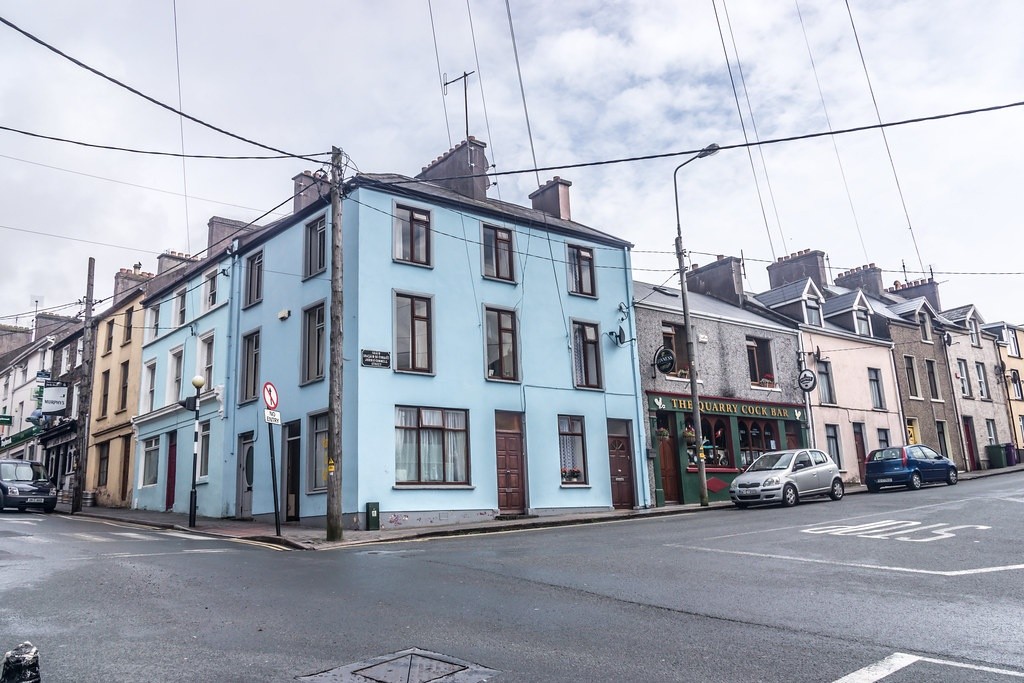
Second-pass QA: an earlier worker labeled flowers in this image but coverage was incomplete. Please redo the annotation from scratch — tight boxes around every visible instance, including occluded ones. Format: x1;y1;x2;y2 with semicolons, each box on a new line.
656;428;669;435
682;425;695;436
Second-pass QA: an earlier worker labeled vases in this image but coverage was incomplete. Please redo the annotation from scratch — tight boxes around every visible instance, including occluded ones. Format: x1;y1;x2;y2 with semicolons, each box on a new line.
657;436;668;442
684;436;695;443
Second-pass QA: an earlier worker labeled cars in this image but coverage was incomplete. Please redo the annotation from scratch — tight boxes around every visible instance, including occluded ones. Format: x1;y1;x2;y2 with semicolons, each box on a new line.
0;460;57;514
864;444;958;494
728;448;844;510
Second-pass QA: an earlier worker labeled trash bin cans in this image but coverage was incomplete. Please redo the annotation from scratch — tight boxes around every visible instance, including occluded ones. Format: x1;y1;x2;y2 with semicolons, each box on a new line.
985;444;1008;468
1003;443;1017;466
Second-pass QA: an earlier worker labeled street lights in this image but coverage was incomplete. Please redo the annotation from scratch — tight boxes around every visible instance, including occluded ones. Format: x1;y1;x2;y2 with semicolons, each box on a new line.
178;375;206;529
673;141;721;507
